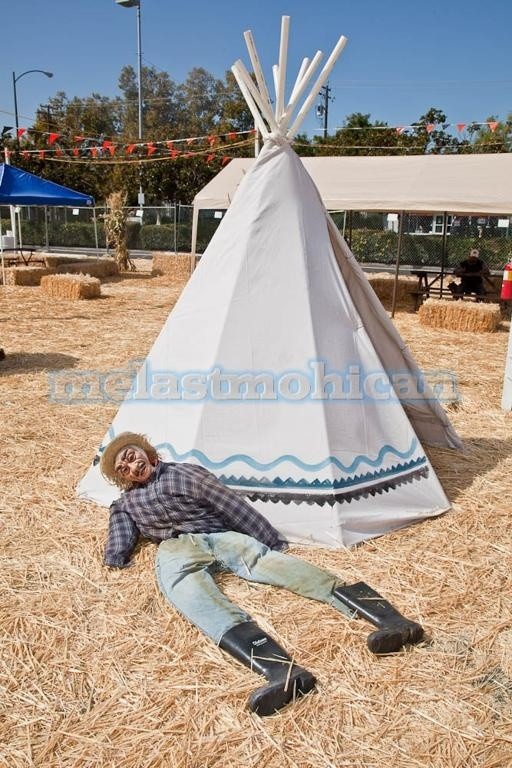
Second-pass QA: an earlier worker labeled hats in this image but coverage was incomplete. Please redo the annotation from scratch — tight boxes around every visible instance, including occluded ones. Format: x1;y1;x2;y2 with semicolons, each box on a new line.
470;249;480;257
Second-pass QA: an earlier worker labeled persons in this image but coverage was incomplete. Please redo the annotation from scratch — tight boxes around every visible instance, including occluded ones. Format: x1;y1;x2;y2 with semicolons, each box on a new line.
453;249;491;303
99;431;424;717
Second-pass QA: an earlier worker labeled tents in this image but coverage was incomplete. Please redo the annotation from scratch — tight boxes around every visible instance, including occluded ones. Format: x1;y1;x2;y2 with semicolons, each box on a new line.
73;134;459;555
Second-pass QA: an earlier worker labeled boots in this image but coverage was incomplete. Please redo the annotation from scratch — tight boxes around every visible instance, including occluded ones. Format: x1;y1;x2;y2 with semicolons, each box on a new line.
218;621;318;720
330;580;425;656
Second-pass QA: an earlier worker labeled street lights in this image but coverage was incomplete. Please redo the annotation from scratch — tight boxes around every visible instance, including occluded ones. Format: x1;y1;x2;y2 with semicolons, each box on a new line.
11;69;53;148
115;0;143;146
